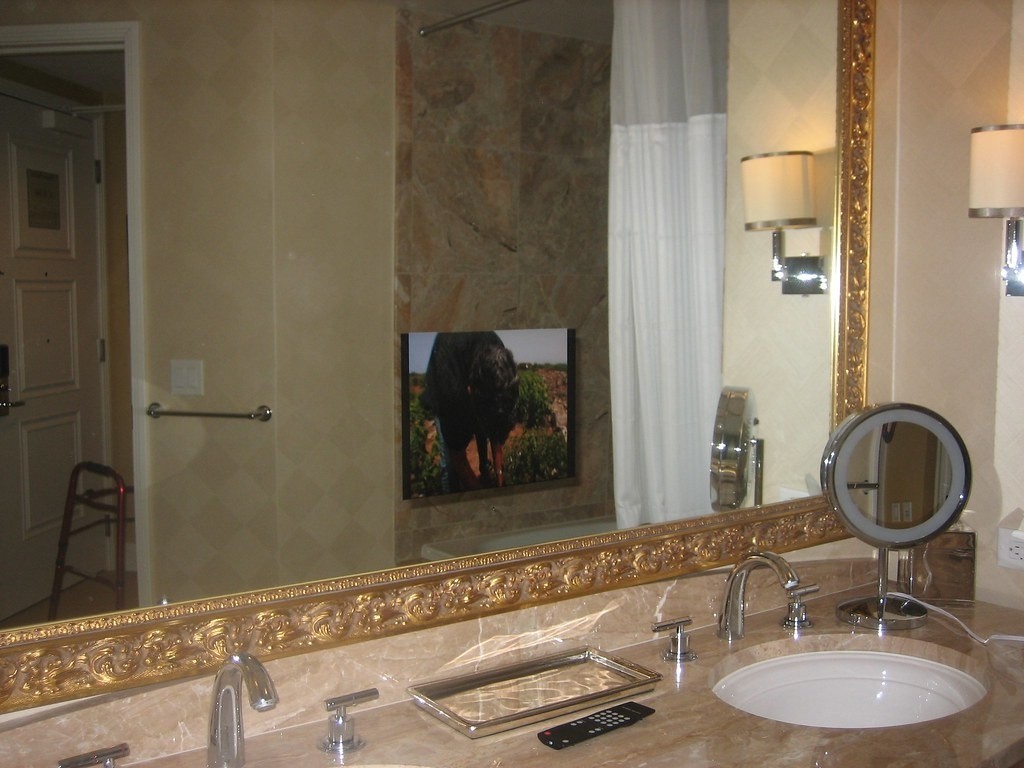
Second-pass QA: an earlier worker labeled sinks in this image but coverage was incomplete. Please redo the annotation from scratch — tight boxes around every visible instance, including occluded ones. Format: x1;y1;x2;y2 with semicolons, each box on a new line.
707;634;992;728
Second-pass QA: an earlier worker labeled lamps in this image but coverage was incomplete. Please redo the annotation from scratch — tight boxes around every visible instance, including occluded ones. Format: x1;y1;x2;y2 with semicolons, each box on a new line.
970;125;1024;297
741;148;837;295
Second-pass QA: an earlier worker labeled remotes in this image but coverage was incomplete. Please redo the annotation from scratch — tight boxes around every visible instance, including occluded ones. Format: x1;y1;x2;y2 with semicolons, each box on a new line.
536;700;655;749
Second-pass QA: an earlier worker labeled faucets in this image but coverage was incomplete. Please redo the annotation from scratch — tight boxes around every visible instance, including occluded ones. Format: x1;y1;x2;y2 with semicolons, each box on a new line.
716;551;800;641
209;652;279;768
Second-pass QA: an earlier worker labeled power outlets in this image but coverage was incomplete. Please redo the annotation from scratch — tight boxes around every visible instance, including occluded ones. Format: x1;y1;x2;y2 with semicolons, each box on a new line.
997;527;1024;568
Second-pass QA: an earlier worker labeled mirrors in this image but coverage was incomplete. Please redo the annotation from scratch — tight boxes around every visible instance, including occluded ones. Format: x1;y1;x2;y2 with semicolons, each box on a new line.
0;4;902;720
